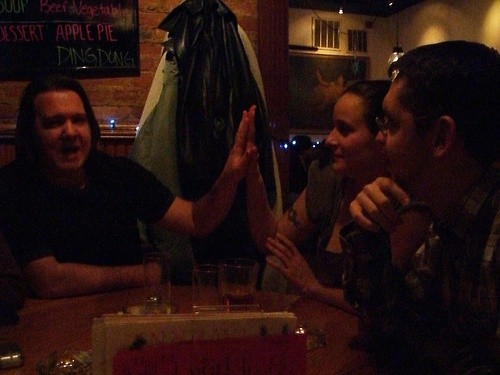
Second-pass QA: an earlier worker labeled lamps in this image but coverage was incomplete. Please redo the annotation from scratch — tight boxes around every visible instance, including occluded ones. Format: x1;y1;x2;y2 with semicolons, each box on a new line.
390;10;406;65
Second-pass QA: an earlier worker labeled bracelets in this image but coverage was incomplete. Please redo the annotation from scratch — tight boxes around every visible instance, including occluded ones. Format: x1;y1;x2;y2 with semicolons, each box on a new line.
142;262;151;287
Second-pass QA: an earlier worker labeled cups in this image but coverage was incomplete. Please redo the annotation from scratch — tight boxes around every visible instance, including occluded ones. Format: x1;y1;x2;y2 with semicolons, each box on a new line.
221;258;258;312
193;264;223;314
141;253;172;314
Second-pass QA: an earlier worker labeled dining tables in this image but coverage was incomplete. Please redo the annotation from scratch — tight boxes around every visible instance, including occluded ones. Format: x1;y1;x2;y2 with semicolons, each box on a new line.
0;286;376;375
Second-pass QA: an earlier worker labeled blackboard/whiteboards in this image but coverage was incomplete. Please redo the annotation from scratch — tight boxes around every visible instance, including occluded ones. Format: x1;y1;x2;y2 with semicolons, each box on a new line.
0;0;141;81
286;50;371;135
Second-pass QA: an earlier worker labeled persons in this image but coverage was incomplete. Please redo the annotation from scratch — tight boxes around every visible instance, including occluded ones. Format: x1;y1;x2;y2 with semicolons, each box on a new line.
338;41;500;375
0;76;252;300
249;80;431;315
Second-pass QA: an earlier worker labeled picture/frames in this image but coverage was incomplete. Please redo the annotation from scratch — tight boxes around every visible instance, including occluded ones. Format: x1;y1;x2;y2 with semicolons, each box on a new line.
289;51;369;134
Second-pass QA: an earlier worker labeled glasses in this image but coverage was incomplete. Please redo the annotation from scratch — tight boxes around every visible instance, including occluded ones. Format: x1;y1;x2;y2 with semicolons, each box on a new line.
374;116;429;135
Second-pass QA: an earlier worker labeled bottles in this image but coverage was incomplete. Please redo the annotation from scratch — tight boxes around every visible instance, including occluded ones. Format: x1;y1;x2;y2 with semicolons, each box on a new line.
38;347;92;375
0;352;24;369
